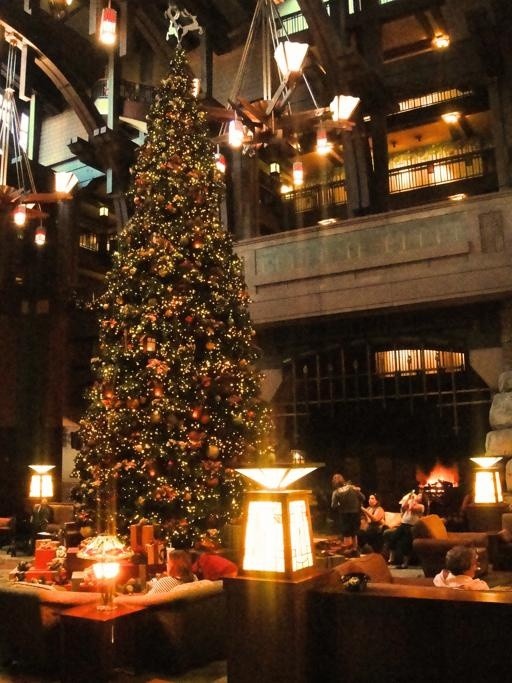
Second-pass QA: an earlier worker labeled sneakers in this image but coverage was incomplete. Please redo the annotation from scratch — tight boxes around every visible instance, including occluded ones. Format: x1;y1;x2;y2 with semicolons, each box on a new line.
387;560;409;570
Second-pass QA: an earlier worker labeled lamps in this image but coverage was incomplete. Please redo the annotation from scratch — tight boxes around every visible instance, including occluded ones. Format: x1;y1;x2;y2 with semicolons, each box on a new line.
28;464;57;502
470;456;507;502
235;463;326;577
206;0;361;187
99;0;119;44
0;41;78;245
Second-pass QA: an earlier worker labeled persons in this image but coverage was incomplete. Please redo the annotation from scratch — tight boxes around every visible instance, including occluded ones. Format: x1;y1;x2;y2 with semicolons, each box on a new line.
431;542;490;590
189;549;240;581
32;497;54;524
382;490;429;569
358;490;386;552
142;548;199;595
68;519;97;547
329;471;365;551
